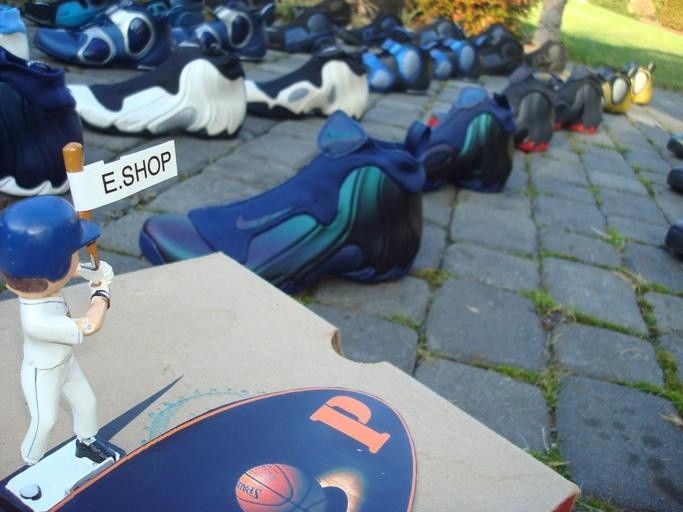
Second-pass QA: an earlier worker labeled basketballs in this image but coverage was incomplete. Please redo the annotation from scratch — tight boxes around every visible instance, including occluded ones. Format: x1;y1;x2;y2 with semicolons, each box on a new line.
233;463;326;511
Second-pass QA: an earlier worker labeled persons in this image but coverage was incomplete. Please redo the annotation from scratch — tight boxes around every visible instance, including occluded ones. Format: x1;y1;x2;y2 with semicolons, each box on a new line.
0;194;128;473
